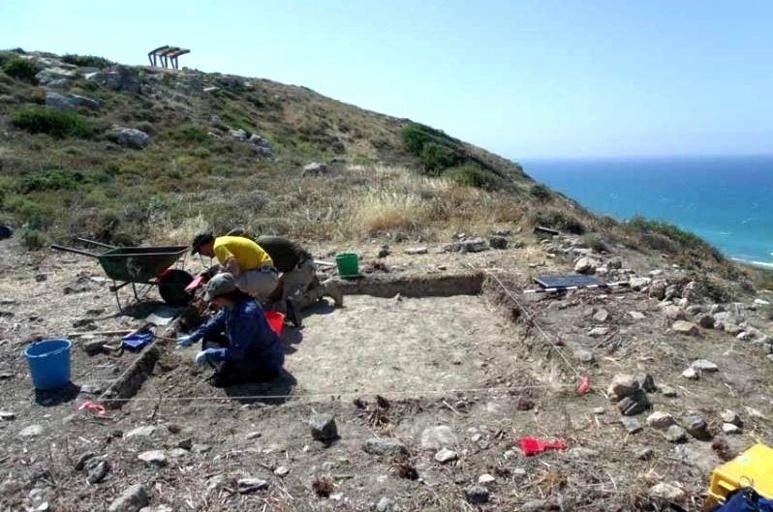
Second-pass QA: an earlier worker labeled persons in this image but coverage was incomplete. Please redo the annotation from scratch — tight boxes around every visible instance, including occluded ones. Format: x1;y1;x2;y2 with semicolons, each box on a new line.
225;228;343;307
176;272;284;388
190;233;302;327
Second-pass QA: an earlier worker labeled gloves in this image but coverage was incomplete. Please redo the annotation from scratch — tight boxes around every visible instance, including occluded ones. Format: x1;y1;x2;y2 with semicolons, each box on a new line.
177;336;193;346
196;351;207;366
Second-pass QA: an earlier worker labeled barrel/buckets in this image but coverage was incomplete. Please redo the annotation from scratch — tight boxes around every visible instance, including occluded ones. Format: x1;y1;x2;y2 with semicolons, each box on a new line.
27;339;72;390
335;252;359;277
264;310;284;335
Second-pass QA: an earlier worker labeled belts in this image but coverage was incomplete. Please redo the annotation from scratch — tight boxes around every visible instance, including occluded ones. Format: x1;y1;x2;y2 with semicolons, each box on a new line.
258;267;278;276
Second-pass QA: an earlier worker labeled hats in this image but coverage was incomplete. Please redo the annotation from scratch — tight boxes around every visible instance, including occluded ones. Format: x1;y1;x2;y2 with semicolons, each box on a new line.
191;233;210;255
203;272;235;302
226;228;243;236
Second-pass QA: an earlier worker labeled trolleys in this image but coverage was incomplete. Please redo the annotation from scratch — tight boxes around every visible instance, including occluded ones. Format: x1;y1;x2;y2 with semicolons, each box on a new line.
50;236;195;306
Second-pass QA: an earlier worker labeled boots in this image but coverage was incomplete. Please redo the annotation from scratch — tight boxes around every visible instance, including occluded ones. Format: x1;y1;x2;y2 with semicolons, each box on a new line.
308;275;342;305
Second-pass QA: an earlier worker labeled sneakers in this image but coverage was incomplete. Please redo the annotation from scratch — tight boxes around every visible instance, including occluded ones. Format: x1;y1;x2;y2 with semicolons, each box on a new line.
285;297;301;326
